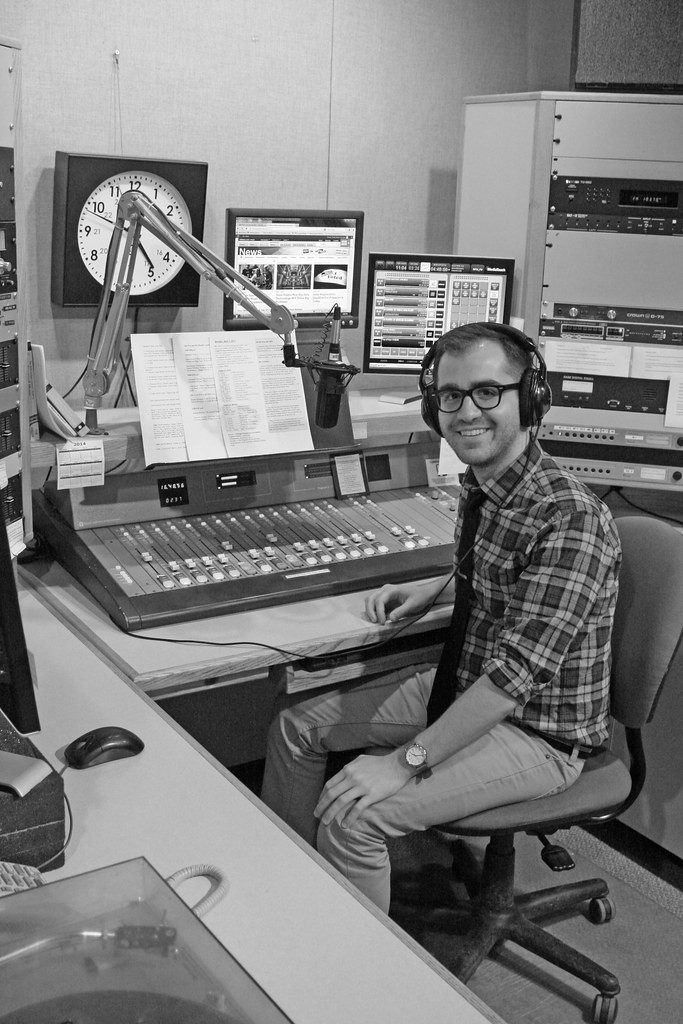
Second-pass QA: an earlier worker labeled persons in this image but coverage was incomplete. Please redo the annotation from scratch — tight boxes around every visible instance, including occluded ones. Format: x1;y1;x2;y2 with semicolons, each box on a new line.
260;323;622;915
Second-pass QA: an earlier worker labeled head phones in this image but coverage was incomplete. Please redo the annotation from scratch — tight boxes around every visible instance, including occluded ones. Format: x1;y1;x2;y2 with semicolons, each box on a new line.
417;321;554;439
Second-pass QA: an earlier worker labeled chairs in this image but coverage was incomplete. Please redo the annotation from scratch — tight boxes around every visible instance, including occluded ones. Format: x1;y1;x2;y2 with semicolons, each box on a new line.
364;516;683;1024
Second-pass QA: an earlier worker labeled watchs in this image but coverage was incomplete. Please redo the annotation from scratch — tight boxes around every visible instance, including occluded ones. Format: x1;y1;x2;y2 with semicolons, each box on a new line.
405;739;429;773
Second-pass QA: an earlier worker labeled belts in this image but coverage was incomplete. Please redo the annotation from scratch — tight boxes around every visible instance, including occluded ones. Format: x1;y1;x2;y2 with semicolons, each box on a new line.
526;725;593;760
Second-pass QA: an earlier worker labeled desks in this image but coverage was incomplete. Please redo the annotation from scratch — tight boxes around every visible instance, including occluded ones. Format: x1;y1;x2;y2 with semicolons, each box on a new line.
0;558;505;1023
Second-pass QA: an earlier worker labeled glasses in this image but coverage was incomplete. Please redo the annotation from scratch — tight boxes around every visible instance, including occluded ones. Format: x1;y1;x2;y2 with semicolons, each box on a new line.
432;383;520;413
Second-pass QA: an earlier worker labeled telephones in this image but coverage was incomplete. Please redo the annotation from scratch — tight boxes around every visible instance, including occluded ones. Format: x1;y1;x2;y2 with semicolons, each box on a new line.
0;861;83;964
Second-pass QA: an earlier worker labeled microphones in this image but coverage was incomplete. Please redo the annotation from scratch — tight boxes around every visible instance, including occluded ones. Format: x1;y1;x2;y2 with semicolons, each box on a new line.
315;306;342;428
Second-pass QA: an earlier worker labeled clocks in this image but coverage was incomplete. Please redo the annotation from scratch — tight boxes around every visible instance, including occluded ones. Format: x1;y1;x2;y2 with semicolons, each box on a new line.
51;150;208;309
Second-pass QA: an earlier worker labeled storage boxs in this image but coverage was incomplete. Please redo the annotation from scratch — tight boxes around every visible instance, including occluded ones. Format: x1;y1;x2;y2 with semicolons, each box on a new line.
0;710;66;873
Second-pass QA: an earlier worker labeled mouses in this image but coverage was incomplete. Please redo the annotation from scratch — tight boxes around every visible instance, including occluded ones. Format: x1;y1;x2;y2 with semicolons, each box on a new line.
64;726;143;769
